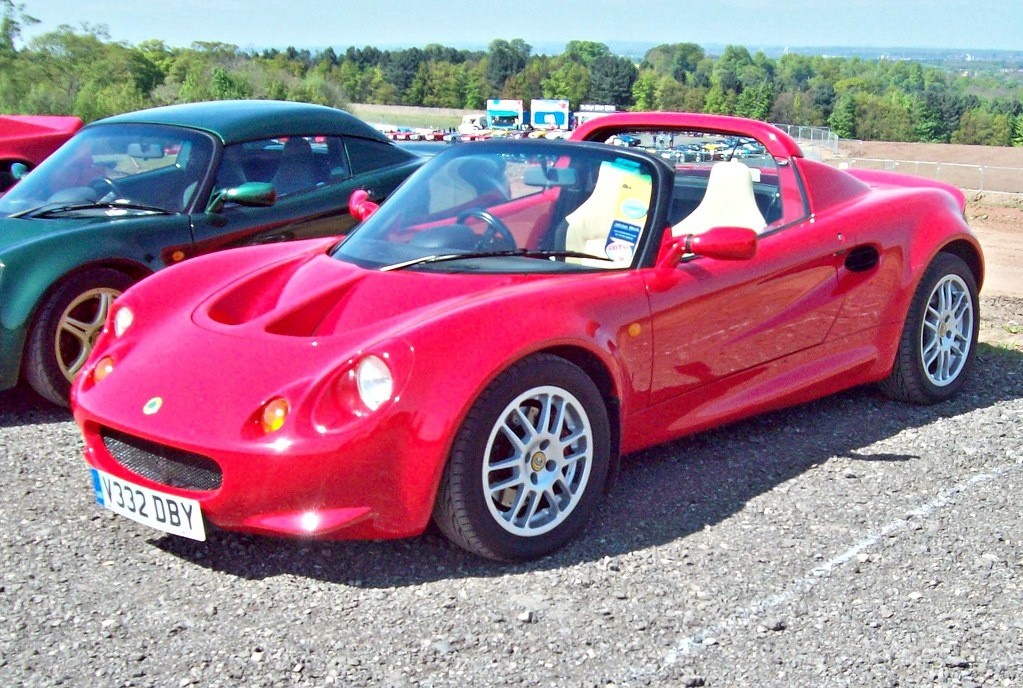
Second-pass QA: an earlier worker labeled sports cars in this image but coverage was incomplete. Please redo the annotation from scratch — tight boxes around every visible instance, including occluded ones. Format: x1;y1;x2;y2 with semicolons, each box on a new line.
70;107;986;567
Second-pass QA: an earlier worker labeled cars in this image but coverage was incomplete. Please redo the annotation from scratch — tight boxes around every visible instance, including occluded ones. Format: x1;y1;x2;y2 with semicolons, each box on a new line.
0;112;105;201
152;111;769;165
0;97;508;418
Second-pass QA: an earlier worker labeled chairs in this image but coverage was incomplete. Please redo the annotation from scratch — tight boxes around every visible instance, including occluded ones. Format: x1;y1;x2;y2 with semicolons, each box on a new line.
269;136;316;196
187;141;248;191
552;160;654;270
669;161;769;257
